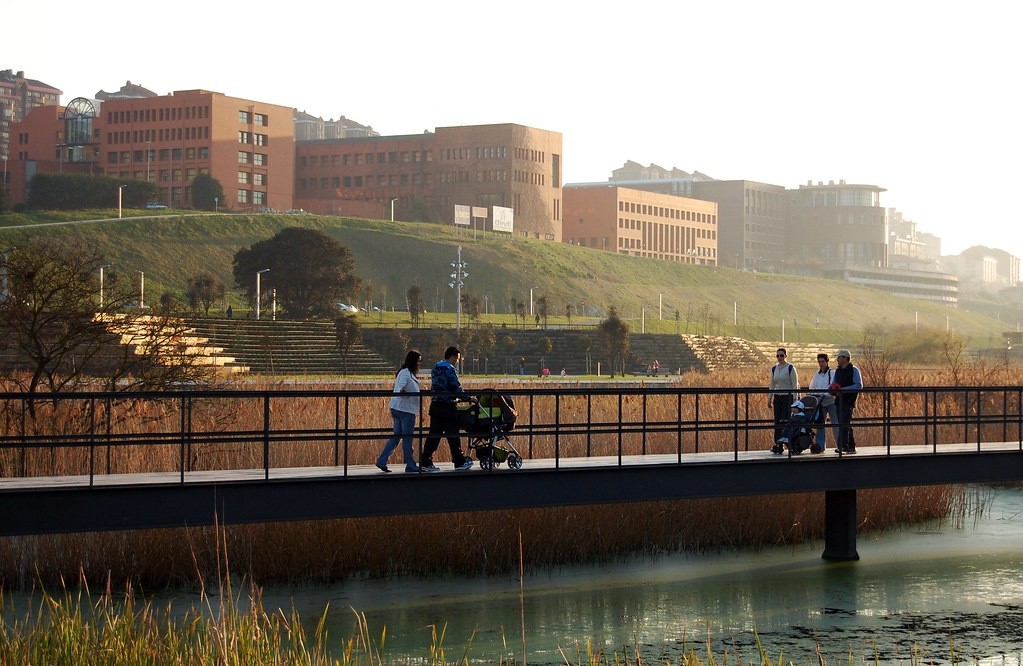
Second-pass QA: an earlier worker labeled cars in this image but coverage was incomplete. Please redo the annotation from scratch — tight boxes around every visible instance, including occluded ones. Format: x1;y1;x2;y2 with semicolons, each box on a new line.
336;303;356;311
147;203;168;209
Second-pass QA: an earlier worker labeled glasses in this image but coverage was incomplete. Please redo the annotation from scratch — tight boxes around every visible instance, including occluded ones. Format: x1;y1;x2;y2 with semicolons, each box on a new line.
776;354;784;357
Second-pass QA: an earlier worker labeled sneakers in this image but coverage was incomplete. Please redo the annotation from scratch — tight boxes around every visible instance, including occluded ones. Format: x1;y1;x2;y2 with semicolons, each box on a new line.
454;462;474;470
778;438;788;443
420;462;439;471
835;447;856;454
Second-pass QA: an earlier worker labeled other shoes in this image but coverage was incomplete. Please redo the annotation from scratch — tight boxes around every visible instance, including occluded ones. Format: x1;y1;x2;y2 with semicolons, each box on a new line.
405;467;422;472
375;462;392;473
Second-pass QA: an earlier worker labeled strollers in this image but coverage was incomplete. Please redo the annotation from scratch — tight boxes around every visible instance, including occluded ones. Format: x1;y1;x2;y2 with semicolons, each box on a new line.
772;393;821;456
455;388;523;470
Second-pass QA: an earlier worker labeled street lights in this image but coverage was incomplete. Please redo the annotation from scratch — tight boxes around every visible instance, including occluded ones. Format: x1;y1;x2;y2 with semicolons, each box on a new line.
448;245;469;373
135;270;144;308
391;198;399;222
78;145;94;175
256;269;270;320
119;185;127;218
530;286;538;315
659;291;668;320
55;143;67;175
100;264;111;307
146;141;150;182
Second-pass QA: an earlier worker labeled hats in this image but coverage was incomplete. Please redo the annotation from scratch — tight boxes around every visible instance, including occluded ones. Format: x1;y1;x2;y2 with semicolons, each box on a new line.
833;349;851;357
791;401;805;410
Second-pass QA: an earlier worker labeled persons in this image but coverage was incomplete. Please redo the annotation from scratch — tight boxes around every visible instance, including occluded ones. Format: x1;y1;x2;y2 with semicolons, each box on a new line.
537;357;545;380
768;348;798;452
561;368;567;378
419;345;478;471
809;353;839;452
827;350;863;454
227;305;232;319
479;399;502;418
647;360;659;376
519;357;525;374
375;349;421;472
